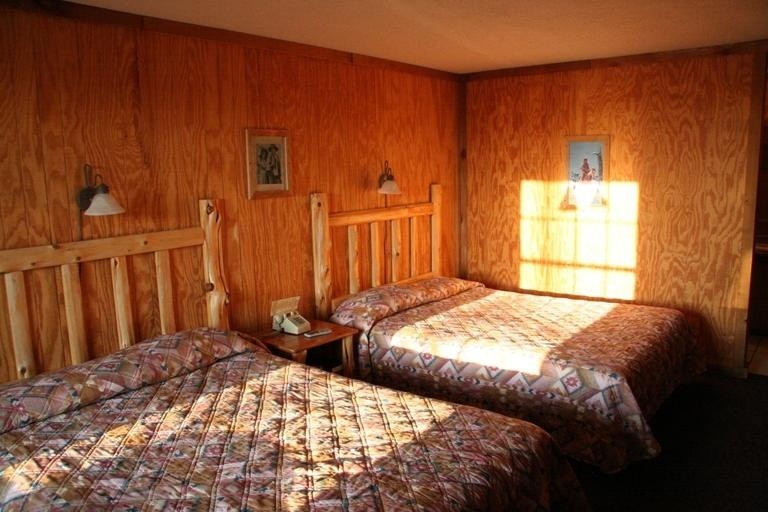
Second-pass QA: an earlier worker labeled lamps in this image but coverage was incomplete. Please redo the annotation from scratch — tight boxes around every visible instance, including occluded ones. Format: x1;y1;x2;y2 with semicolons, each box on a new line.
378;159;402;210
75;164;125;238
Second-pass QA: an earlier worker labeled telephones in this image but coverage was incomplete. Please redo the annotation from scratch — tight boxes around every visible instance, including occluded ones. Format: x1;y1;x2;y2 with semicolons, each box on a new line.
272;309;311;335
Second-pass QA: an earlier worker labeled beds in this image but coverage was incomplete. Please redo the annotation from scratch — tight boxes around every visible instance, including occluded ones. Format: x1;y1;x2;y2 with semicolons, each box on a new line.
308;185;709;478
1;199;556;510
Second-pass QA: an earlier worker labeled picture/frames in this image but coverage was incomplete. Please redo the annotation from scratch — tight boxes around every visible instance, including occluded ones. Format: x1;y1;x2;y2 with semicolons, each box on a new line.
563;134;610;182
245;127;294;200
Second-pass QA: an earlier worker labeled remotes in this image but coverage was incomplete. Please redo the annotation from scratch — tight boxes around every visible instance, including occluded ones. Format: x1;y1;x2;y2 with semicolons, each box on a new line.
303;328;332;338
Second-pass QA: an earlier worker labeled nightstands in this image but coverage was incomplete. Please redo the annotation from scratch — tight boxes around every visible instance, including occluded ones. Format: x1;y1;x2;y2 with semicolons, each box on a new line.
257;318;360;378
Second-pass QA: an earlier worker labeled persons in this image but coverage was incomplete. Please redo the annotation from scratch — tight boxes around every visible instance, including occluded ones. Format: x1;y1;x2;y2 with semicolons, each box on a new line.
257;148;268;184
266;144;281;184
578;159;589;182
582;168;596;184
